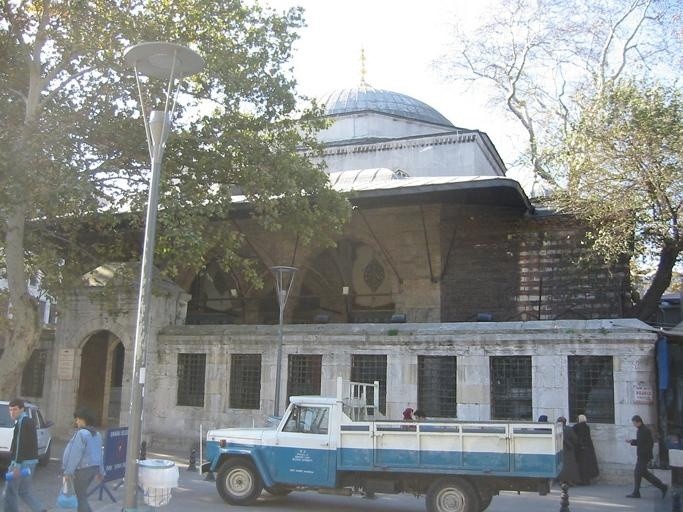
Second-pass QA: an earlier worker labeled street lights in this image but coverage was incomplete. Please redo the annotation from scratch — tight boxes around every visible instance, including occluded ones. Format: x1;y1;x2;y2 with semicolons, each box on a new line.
112;37;208;512
266;263;298;419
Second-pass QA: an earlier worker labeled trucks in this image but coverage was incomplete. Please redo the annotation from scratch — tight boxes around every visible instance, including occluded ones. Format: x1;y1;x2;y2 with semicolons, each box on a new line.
198;369;567;510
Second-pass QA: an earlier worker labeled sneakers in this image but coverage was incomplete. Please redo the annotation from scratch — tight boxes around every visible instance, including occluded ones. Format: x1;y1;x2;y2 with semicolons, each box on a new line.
626;493;641;498
662;484;667;498
558;478;590;487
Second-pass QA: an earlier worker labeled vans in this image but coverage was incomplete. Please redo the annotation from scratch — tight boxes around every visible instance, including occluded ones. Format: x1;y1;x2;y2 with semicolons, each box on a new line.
0;398;55;472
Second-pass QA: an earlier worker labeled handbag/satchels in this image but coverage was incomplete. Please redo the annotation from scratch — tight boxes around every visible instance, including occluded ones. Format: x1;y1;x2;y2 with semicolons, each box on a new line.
55;475;78;509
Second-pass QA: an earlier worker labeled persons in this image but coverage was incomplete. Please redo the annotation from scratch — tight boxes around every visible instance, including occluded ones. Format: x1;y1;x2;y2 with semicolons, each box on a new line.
537;414;549;423
401;408;416;429
625;414;668;500
414;410;429;421
557;417;580;487
3;398;46;512
571;415;600;486
62;404;106;511
